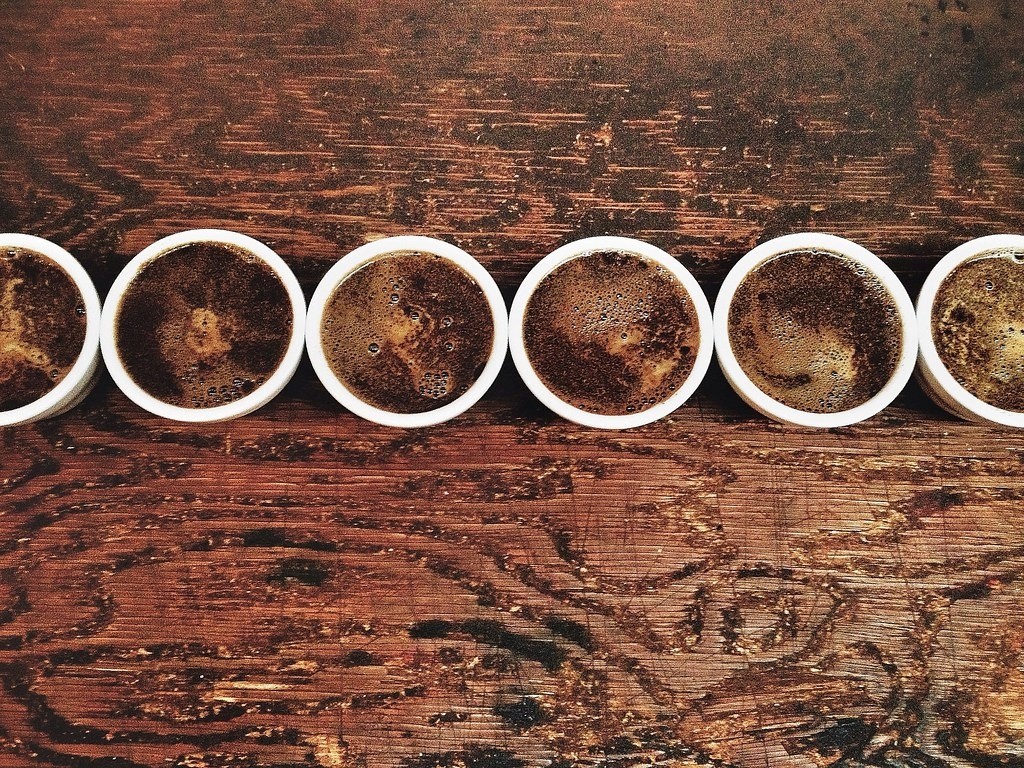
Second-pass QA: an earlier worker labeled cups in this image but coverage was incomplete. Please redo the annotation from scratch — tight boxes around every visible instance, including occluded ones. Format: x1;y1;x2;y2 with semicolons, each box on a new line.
916;234;1024;429
714;233;919;428
0;232;101;427
305;235;507;430
101;227;306;426
508;236;714;431
